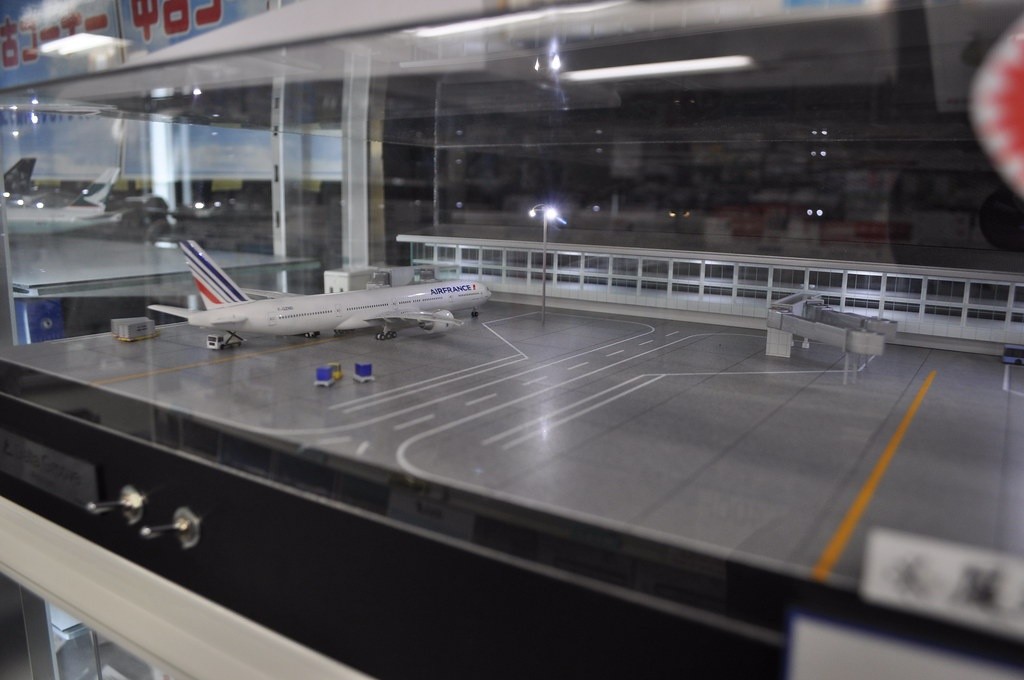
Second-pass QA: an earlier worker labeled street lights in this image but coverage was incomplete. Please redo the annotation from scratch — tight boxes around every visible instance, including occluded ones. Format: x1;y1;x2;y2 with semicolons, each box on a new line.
528;203;557;322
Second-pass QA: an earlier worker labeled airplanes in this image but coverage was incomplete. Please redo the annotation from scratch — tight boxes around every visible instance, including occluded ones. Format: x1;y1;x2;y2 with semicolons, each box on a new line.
148;239;494;351
